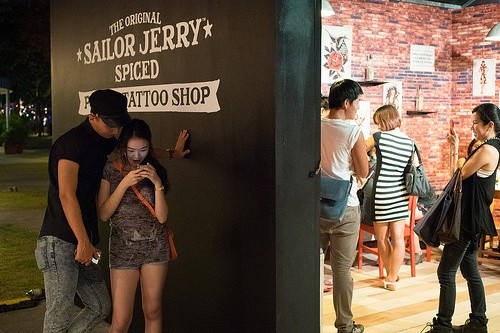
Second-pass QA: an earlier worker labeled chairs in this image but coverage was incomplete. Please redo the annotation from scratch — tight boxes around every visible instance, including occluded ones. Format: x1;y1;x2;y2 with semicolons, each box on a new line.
478;190;500;268
358;195;418;279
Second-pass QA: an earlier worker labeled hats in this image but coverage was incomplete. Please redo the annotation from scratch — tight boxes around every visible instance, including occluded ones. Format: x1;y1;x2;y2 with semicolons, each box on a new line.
89;89;131;128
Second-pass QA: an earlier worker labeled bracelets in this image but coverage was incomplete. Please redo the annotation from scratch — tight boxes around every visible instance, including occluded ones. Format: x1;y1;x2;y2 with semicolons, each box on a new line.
451;152;458;156
156;186;164;191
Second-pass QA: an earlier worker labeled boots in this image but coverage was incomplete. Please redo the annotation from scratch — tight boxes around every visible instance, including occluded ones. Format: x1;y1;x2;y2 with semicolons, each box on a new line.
452;313;488;333
420;317;453;333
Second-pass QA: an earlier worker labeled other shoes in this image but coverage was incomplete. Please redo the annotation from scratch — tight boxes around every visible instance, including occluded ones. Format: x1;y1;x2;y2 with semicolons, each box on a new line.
352;324;364;333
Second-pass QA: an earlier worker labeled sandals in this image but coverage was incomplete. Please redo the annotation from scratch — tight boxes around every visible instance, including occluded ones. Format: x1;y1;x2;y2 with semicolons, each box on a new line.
396;276;399;282
371;279;396;291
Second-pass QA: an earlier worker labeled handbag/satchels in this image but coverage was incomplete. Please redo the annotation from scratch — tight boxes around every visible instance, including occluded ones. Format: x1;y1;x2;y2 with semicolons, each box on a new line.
436;168;463;245
320;177;352;222
413;168;459;247
164;223;177;258
405;140;430;196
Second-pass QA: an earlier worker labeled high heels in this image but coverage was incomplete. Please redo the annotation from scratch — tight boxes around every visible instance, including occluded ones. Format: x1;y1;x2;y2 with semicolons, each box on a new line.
406;253;423;265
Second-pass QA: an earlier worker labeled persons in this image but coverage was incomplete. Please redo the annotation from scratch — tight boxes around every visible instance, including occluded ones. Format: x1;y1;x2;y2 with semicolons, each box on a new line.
321;95;334;291
365;105;415;290
321;79;369;333
98;118;171;333
34;89;190;333
425;103;500;333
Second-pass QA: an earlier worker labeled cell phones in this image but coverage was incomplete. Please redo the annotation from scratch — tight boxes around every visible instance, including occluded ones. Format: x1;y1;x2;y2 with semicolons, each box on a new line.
450;119;454;136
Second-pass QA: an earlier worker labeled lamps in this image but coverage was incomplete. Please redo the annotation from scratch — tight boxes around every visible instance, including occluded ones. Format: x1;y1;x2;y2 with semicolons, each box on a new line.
321;0;335;18
485;22;500;41
